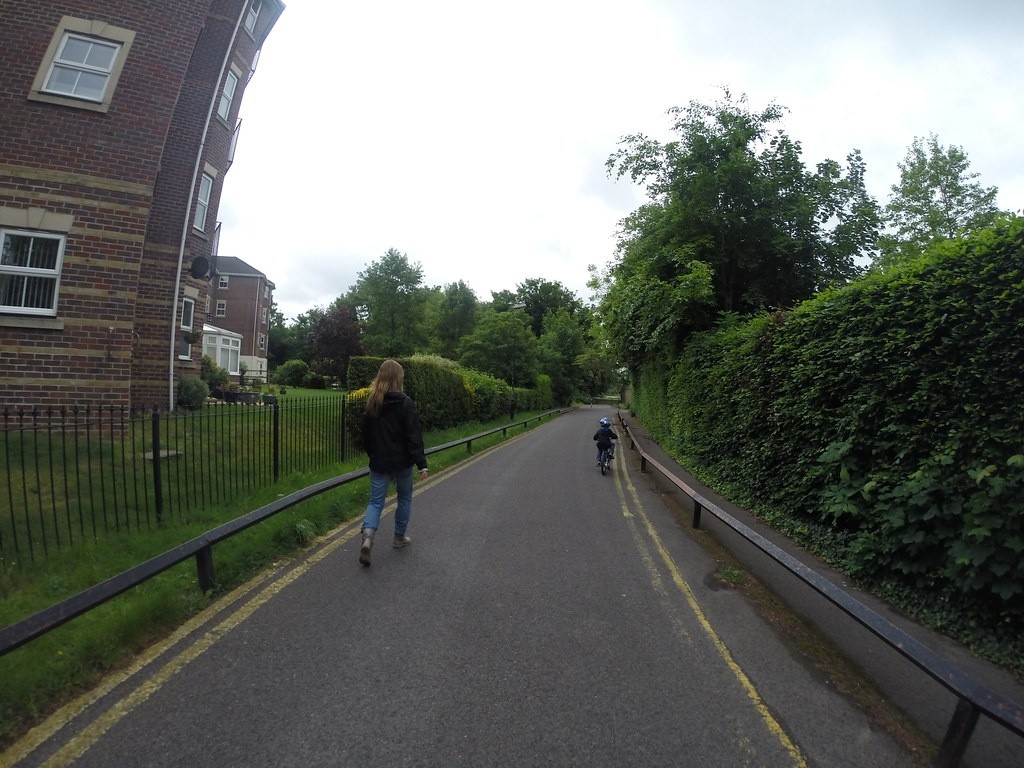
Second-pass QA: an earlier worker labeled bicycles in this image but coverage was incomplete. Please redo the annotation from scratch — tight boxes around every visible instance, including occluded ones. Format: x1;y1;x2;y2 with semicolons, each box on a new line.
595;436;618;476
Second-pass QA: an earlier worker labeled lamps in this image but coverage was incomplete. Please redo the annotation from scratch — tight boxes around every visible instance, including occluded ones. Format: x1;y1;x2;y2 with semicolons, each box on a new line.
206;313;214;323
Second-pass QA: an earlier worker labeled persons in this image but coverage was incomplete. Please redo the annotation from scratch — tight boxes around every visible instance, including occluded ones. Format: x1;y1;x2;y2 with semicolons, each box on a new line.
358;358;431;566
593;416;619;471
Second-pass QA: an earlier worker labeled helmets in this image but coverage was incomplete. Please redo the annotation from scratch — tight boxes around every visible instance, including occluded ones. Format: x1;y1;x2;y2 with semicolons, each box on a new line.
599;417;611;426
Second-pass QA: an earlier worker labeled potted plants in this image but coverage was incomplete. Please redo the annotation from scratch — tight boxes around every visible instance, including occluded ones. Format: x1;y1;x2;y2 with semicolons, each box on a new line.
240;385;260;404
214;368;231;399
262;385;278;404
223;384;240;403
280;384;287;394
178;373;212;411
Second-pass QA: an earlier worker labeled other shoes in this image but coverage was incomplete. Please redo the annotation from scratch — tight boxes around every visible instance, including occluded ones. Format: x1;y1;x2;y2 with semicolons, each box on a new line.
597;461;601;467
609;454;614;459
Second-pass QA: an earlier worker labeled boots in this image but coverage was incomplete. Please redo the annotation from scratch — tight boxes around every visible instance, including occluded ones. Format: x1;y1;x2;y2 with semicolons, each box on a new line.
392;534;411;547
359;528;376;565
